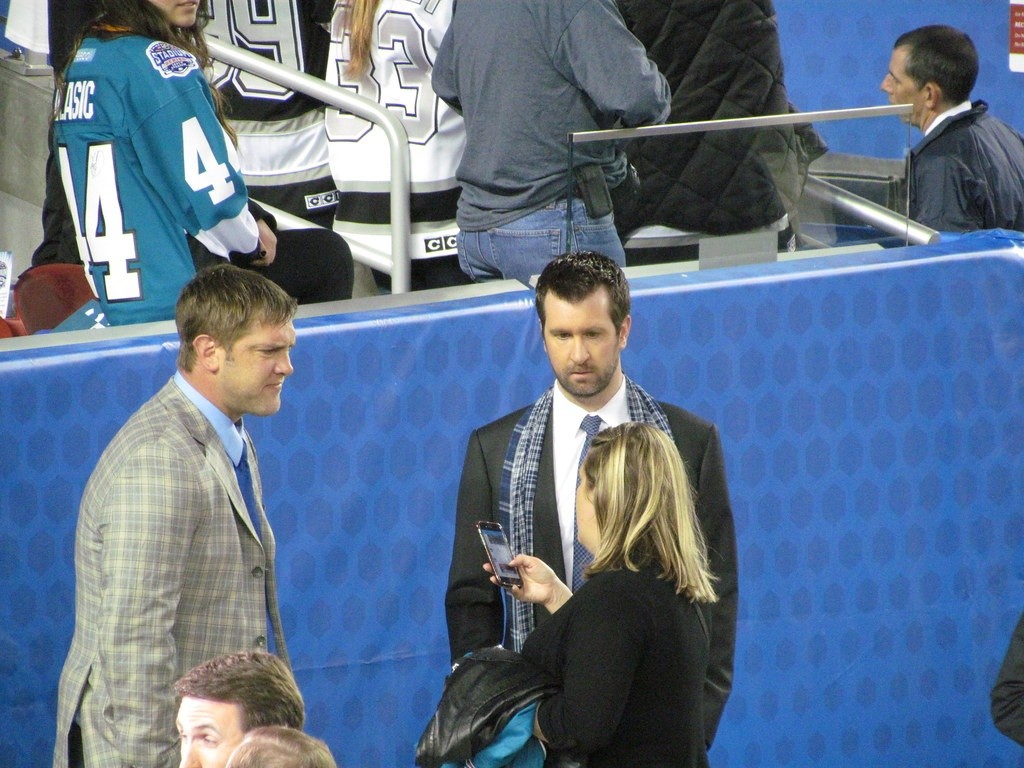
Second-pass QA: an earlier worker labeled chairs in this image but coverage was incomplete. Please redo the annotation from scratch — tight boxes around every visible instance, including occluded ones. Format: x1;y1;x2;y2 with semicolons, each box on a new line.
14;262;96;335
0;316;13;339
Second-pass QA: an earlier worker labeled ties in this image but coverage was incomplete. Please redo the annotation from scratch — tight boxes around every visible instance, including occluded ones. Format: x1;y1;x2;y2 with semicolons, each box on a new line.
573;414;600;595
233;440;277;655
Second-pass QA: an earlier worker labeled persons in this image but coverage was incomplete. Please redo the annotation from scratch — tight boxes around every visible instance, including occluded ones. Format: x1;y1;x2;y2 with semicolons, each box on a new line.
444;249;739;768
880;24;1024;232
32;0;829;338
54;263;337;768
991;611;1024;746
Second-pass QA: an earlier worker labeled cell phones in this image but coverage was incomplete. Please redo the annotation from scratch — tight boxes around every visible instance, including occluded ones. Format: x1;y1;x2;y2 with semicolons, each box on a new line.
477;521;524;588
